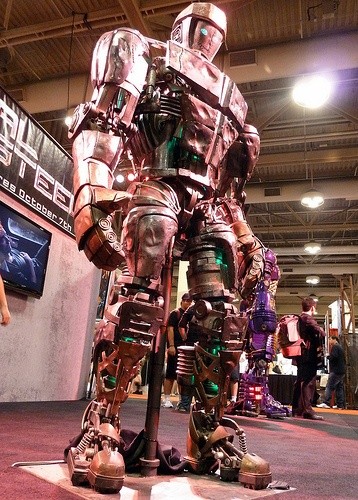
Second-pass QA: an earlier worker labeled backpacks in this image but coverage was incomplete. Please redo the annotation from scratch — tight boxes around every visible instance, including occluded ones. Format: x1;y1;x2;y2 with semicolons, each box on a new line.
278;314;310;357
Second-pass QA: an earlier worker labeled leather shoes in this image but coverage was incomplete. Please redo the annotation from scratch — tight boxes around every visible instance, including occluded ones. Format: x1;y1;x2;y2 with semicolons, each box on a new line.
292;412;325;421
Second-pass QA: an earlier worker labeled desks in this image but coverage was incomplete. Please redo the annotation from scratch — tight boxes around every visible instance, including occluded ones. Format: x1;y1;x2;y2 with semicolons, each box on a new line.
268;374;298;405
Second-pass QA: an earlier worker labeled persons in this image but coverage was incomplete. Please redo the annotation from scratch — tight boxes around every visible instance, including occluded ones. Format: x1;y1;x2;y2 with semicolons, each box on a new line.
0;274;10;325
0;224;45;289
293;298;326;419
64;3;293;493
161;294;254;414
316;335;347;409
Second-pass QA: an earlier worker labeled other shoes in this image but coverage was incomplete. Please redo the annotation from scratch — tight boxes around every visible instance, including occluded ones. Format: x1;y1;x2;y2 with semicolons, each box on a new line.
162;399;173;407
332;405;338;408
316;402;330;408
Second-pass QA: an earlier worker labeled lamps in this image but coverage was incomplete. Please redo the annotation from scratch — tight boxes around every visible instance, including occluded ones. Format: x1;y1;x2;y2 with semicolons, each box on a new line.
291;5;336;109
302;143;325;209
64;108;77;129
306;275;320;284
303;216;321;253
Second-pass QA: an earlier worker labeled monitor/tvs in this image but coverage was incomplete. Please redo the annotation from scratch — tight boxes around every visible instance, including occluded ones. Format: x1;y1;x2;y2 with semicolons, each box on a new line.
0;201;52;299
